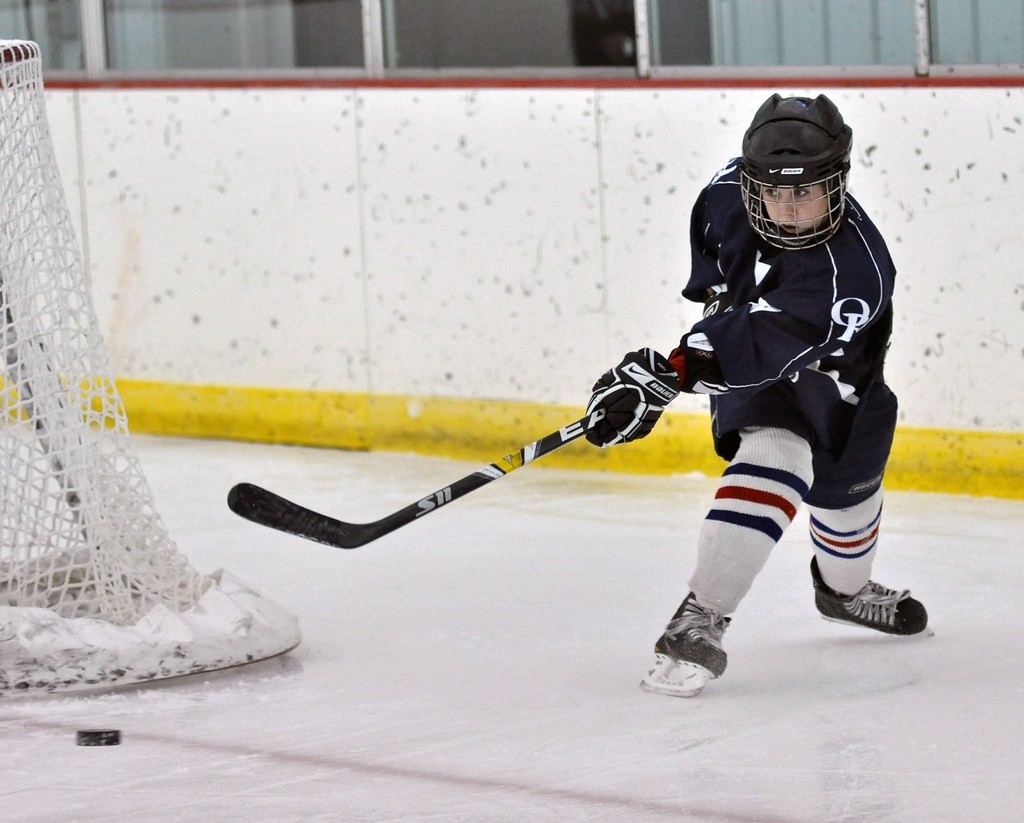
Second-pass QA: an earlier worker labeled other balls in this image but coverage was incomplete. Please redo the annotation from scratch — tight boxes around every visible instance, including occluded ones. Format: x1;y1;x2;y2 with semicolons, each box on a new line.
75;727;121;747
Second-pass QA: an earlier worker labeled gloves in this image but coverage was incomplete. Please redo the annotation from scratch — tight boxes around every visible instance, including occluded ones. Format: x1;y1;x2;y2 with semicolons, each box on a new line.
585;346;680;449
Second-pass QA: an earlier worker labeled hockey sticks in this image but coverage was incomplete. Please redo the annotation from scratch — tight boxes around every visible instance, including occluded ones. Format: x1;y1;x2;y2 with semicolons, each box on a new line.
224;407;608;551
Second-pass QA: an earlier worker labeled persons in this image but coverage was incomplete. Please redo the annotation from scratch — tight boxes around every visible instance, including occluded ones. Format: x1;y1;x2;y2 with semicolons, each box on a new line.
587;93;935;698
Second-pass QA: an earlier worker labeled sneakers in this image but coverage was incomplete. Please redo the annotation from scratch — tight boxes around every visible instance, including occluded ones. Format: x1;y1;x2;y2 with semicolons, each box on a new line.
810;554;934;636
640;592;731;698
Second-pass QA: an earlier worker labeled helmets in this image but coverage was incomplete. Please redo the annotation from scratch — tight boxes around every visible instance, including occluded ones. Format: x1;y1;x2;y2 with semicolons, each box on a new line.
739;94;853;251
